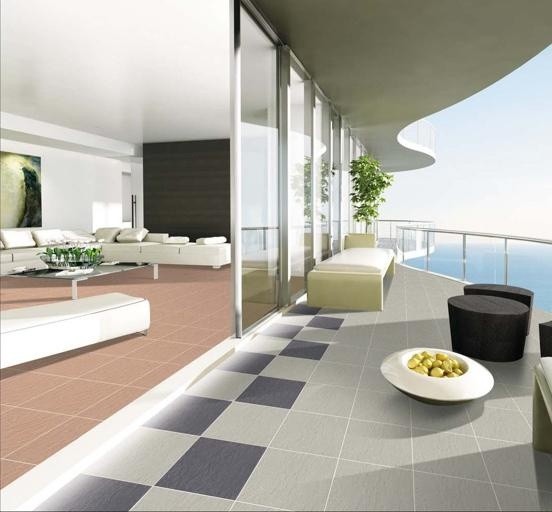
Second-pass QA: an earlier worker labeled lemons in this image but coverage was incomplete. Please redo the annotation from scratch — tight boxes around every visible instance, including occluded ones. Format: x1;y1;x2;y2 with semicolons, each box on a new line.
407;351;463;378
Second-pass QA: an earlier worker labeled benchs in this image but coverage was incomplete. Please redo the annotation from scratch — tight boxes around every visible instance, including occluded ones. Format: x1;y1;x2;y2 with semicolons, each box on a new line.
242;243;330;285
0;292;150;371
306;247;395;311
533;357;552;453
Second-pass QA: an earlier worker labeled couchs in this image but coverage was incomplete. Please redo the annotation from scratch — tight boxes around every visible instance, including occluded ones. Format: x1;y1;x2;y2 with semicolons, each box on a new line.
0;226;232;277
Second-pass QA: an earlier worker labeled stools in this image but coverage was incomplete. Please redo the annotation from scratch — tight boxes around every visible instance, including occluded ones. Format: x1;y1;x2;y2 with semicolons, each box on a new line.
539;321;552;357
464;283;534;335
448;294;530;362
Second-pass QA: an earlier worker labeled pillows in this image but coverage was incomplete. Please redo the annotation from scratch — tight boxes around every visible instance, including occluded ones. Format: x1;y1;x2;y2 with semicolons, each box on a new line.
96;227;120;243
31;229;66;247
196;236;227;245
164;236;189;244
0;240;4;249
116;228;149;242
62;228;95;243
0;229;36;249
